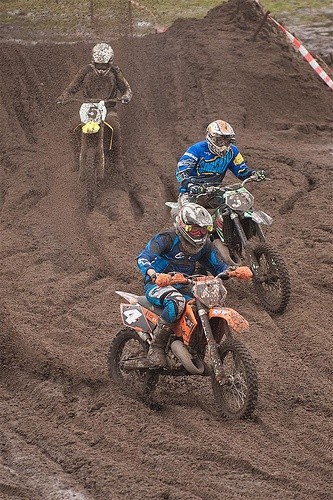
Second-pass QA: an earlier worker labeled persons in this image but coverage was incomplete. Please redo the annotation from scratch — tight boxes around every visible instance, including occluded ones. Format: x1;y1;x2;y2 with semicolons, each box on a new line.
175;119;266;216
136;203;253;373
57;43;133;175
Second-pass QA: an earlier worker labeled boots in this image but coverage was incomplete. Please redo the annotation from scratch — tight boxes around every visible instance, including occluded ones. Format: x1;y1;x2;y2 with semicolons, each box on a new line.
70;143;81;172
113;147;125;172
147;316;175;368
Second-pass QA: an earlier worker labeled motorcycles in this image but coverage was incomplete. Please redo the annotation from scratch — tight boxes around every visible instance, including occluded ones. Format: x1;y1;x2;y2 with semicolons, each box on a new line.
107;264;259;420
56;97;123;209
163;169;292;314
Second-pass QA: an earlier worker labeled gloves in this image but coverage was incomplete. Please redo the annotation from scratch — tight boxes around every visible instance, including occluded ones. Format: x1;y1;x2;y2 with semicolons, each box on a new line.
57;95;67;105
189;184;204;194
236;266;253;281
150;272;172;287
254;170;266;180
121;95;130;104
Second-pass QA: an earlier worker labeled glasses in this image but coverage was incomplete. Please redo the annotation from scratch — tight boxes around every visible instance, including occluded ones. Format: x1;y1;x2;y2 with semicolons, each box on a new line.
185;224;209;236
213;136;234;147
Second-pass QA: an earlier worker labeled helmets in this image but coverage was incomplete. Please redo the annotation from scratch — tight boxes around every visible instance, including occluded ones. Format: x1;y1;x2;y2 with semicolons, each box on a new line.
91;42;114;76
206;119;235;158
174;203;214;248
